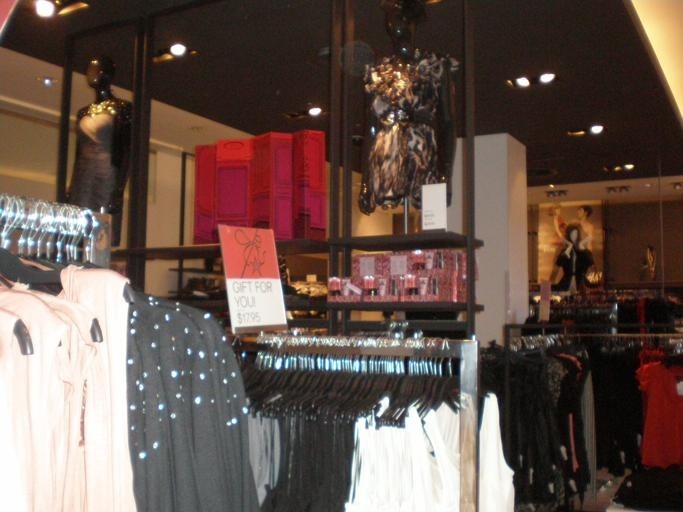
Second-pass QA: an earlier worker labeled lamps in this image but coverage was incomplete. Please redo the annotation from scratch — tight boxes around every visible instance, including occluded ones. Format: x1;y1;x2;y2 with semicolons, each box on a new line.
291;101;320;124
670;182;682;191
513;69;556;87
152;48;191;68
547;190;569;200
608;160;637;178
36;0;86;19
567;122;607;143
606;184;629;195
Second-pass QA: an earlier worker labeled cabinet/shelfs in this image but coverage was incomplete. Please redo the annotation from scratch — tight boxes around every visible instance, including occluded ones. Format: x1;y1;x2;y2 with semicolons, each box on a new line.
140;0;330;326
330;2;484;335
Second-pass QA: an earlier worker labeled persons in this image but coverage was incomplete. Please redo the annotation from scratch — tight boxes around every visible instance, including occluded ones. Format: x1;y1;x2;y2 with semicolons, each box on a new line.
356;0;458;216
550;205;596;291
68;55;132;249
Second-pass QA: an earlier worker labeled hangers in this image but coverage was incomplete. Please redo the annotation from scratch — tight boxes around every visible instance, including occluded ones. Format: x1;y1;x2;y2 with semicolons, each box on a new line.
588;336;683;365
232;325;461;430
0;196;101;295
554;290;670;313
505;330;577;356
